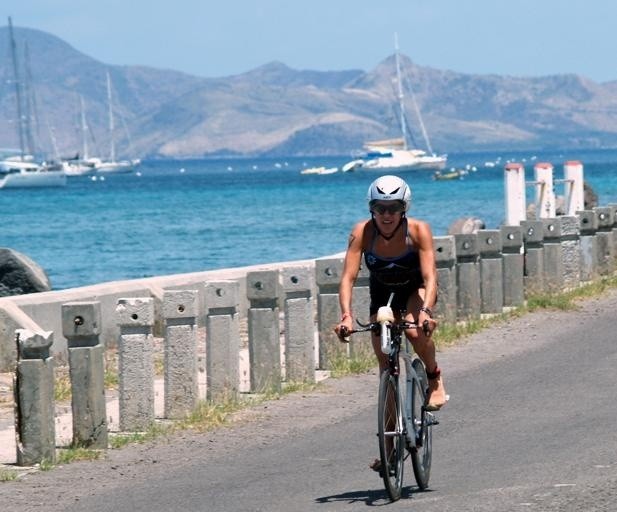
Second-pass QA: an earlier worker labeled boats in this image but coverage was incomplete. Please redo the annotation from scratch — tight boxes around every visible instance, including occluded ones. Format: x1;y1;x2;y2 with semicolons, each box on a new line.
300;166;337;176
436;171;466;180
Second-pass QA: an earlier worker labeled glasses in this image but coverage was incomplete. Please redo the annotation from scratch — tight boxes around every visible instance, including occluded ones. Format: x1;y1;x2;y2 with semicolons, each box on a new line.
371;203;404;215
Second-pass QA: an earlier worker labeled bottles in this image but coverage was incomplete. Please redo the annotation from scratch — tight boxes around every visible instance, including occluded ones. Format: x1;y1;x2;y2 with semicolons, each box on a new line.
376;306;394;353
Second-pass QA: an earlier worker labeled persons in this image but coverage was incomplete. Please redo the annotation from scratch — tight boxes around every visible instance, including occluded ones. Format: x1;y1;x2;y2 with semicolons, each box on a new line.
329;170;451;473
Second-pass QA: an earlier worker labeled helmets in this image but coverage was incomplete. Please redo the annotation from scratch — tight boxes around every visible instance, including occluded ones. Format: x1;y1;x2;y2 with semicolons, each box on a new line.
368;175;411;211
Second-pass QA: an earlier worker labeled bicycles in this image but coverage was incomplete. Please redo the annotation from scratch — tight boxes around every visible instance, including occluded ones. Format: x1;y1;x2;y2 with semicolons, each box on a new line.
340;312;439;500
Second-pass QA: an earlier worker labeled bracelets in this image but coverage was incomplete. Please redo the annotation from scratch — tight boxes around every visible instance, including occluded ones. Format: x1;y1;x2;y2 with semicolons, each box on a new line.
420;306;433;319
341;311;352;321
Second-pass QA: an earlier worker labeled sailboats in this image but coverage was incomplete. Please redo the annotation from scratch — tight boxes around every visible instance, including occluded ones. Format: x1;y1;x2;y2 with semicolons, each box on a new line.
0;12;142;187
344;27;448;174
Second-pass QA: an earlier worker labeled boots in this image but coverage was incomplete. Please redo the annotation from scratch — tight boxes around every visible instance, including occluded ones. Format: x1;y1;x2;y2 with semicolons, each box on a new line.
426;366;446;407
